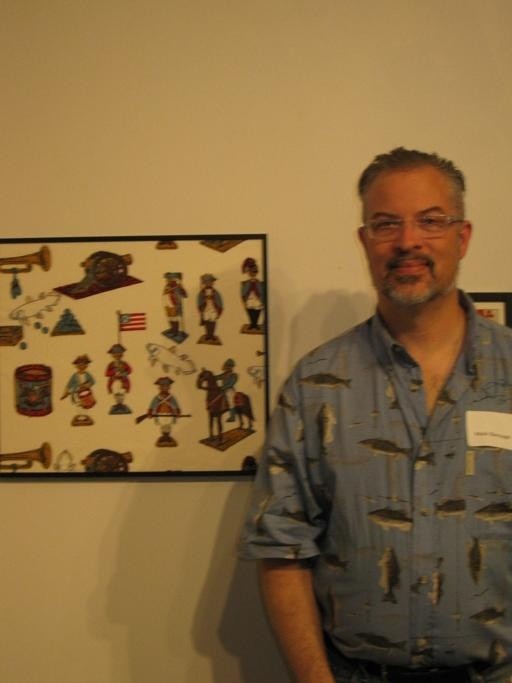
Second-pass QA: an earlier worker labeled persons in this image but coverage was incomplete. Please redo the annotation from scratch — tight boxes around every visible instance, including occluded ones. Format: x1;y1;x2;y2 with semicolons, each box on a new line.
161;271;188;336
238;143;512;682
65;352;97;417
238;256;267;331
148;376;182;444
195;273;223;342
215;358;240;424
104;342;131;410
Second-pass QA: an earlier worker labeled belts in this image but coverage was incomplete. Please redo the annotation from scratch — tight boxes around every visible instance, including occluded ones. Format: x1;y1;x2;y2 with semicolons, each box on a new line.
326;633;491;683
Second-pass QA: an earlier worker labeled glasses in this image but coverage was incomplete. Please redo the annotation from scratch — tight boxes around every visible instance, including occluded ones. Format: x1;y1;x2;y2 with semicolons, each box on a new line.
365;215;465;243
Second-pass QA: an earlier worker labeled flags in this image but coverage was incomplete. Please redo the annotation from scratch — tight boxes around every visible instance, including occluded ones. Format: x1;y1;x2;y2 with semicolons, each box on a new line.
120;312;147;332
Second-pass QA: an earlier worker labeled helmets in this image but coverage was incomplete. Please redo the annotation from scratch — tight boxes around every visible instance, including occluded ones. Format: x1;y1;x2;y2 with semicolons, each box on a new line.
241;258;258;274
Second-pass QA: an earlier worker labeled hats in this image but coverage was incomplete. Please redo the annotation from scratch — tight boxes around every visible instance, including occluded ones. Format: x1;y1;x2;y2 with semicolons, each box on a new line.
72;355;93;362
155;378;175;385
199;275;219;283
107;345;125;354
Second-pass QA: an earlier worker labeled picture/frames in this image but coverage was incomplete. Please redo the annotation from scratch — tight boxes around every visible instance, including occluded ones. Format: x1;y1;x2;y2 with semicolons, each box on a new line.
469;294;512;327
0;231;271;482
